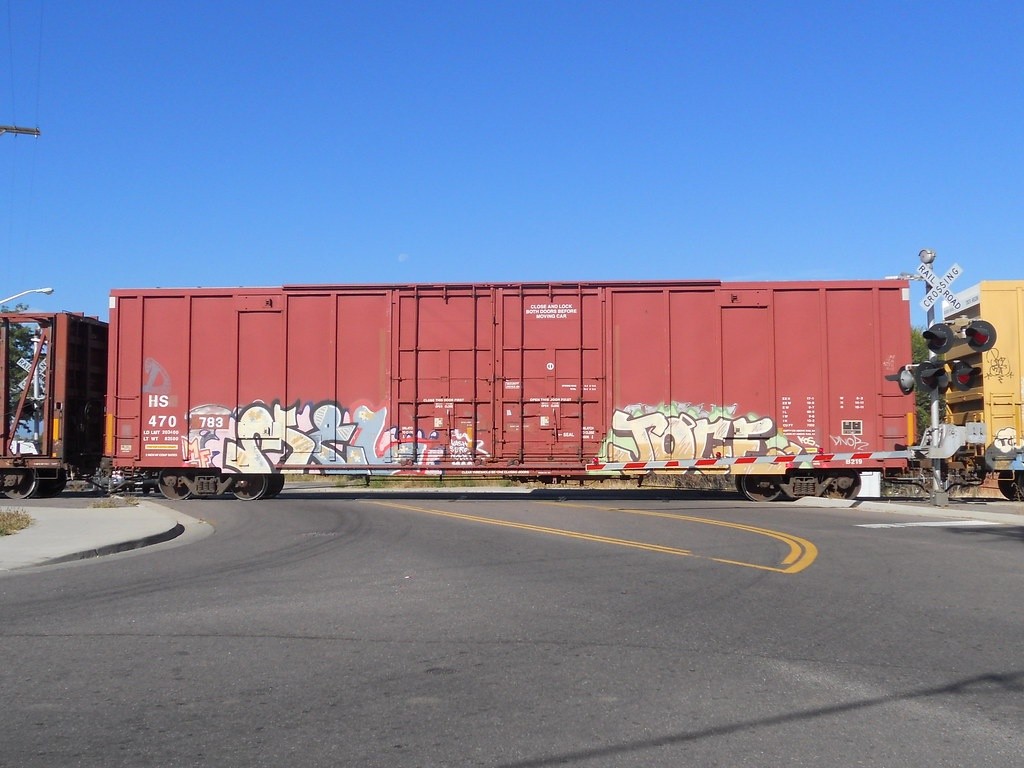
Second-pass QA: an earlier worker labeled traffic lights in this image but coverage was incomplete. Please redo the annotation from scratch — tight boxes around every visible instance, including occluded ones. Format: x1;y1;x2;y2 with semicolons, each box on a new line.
965;321;997;353
952;361;981;392
922;323;953;354
915;363;945;392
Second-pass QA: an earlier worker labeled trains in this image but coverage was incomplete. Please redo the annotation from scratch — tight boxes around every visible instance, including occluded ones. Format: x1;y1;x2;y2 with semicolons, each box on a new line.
1;279;1023;502
